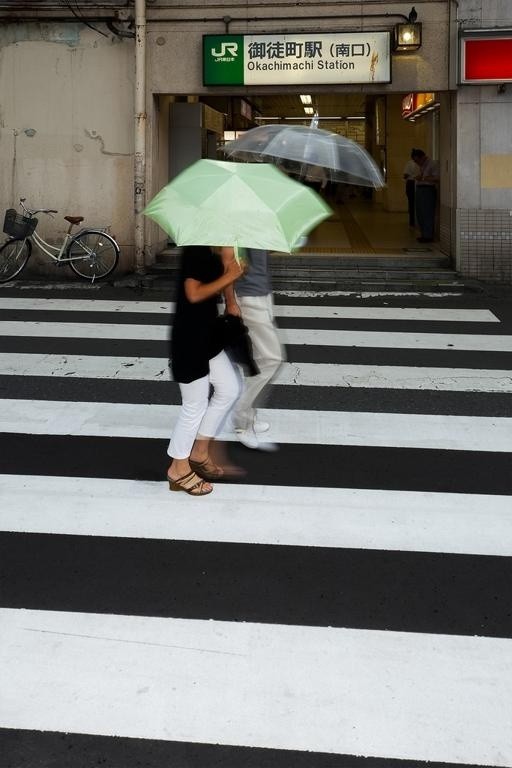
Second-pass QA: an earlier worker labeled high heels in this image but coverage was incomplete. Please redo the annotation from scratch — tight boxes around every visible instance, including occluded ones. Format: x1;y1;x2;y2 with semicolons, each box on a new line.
167;456;224;496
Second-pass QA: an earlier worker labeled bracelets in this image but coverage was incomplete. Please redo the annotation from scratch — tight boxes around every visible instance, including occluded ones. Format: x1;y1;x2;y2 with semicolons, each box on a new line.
421;176;425;180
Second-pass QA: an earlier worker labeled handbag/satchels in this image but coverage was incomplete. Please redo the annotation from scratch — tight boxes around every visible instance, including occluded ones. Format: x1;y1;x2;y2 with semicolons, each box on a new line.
218;314;261;378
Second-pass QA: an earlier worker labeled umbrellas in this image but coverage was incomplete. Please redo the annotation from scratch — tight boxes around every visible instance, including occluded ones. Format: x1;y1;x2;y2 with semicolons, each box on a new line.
217;111;386;190
143;158;333;254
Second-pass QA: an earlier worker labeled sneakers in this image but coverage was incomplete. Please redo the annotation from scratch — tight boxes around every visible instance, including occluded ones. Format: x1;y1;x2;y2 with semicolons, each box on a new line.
227;412;270;449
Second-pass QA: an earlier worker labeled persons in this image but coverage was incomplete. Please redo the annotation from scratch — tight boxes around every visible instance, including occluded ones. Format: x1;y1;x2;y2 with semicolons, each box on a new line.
277;159;373;209
402;160;422;232
219;247;286;449
165;246;245;495
411;150;440;243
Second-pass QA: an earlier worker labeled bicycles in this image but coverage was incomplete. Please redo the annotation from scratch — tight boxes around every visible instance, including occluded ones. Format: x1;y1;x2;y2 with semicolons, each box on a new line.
0;199;120;286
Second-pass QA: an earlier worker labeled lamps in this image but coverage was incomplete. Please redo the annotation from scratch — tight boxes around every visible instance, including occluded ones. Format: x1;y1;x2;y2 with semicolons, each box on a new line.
392;21;422;54
